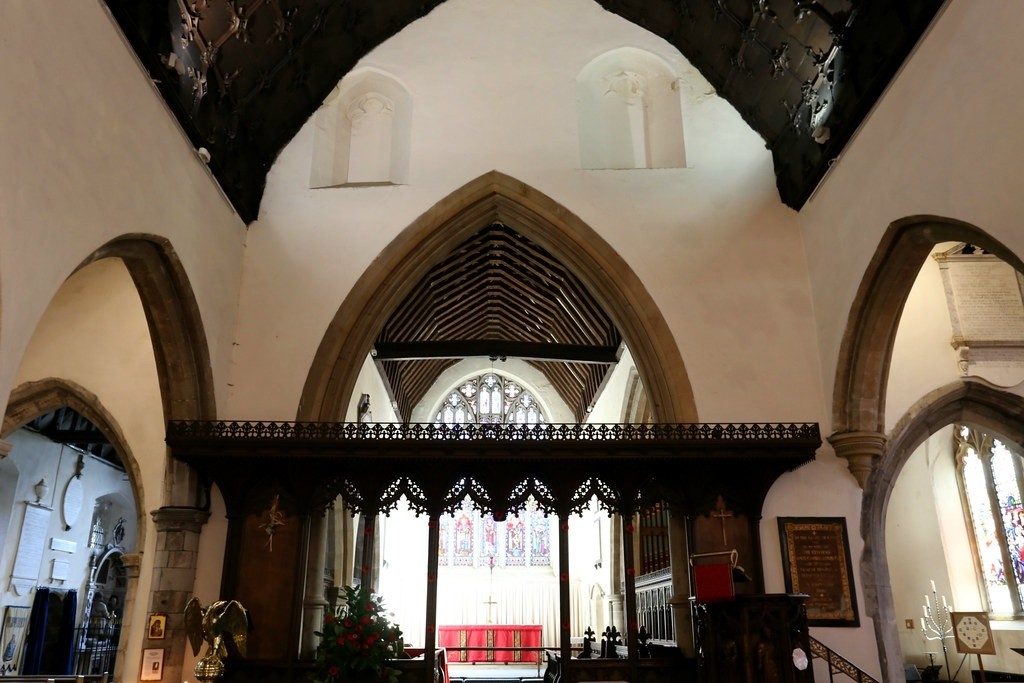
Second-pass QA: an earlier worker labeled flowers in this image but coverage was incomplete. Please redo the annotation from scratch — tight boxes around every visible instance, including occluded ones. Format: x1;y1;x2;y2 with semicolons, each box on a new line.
312;584;407;683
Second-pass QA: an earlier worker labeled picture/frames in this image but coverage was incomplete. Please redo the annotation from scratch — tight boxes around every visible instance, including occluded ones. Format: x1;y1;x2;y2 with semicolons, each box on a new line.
147;616;167;640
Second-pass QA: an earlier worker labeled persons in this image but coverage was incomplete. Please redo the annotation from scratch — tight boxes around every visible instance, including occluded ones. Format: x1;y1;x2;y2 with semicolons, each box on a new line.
88;592;121;640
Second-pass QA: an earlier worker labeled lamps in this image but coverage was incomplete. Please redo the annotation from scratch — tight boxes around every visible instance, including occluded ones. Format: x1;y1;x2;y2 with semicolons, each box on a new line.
35;478;49;503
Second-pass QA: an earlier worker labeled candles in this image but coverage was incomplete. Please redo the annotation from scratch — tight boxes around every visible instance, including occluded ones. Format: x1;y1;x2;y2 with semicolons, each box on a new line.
921;580;953;631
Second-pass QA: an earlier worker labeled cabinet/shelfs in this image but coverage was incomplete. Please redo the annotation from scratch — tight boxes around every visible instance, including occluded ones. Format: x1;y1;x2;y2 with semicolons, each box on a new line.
693;593;814;683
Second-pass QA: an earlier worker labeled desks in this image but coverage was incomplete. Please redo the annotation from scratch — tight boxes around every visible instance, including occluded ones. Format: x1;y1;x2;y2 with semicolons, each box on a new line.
437;625;544;665
